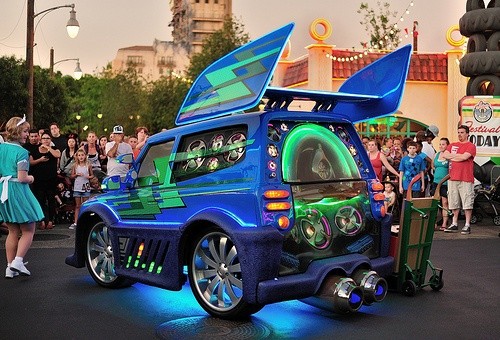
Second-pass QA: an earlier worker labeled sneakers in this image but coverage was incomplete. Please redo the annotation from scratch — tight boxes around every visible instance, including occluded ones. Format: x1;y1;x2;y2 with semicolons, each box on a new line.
5;268;19;278
9;260;31;276
461;226;471;234
443;224;459;233
69;223;77;230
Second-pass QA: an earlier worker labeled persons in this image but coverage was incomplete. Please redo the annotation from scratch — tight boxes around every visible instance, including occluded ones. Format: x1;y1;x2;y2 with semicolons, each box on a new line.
0;115;44;278
432;138;449;231
363;126;439;218
0;124;168;230
441;125;476;234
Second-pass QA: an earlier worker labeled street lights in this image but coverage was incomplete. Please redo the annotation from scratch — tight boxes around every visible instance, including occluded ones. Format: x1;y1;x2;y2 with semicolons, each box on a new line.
26;0;81;131
49;49;83;81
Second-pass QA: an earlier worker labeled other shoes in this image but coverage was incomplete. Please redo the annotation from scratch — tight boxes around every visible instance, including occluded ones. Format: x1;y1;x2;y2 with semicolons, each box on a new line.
46;223;53;229
39;224;46;230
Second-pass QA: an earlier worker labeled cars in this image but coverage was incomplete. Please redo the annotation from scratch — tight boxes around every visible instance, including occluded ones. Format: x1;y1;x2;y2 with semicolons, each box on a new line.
65;110;396;316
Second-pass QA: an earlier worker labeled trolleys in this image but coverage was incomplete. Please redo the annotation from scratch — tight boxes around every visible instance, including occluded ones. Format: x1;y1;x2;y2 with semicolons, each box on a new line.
386;171;451;296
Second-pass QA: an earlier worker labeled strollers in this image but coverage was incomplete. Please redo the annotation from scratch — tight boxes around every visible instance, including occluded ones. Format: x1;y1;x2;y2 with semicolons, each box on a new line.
469;176;500;226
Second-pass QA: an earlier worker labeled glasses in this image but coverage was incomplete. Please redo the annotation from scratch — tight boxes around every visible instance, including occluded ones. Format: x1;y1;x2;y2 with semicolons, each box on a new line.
41;137;51;139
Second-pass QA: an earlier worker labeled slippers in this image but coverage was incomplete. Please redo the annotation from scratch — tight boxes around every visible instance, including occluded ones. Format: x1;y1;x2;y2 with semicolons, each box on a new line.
439;227;447;231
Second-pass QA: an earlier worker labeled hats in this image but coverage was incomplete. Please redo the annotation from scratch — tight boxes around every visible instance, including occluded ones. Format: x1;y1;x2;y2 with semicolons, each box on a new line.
428;125;440;138
112;125;124;134
